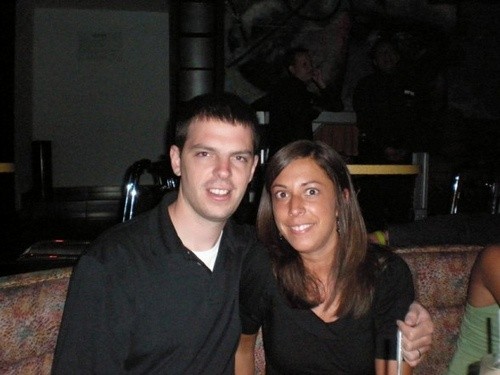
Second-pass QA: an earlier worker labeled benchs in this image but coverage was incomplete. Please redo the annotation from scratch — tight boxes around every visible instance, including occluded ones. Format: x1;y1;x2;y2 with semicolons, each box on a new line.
0;243;483;375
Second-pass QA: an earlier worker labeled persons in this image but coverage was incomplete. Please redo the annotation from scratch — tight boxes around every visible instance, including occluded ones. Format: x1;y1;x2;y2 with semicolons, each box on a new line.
251;30;500;236
229;141;417;374
49;89;435;374
445;242;499;374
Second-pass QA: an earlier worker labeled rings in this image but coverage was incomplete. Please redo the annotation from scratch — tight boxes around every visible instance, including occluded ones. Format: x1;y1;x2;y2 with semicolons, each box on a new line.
416;349;422;359
